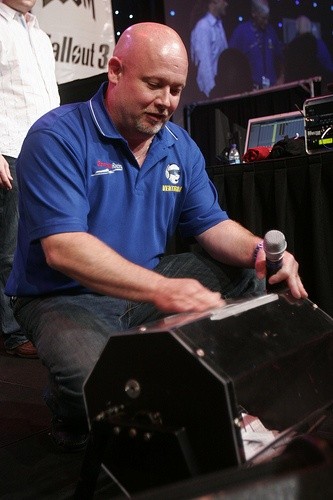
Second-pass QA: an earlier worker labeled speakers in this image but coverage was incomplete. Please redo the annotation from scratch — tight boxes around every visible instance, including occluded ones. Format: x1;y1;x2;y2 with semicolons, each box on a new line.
58;72;109;106
117;440;333;500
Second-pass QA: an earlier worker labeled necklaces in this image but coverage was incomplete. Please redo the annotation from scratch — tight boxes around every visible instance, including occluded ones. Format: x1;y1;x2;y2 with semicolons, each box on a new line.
134;136;155;160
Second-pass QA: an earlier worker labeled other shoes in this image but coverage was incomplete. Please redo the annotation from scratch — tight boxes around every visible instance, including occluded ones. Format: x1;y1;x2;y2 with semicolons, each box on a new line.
6;340;39;357
45;386;90;451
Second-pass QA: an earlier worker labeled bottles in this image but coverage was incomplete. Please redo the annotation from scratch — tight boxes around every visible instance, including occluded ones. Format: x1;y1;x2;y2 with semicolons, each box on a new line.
228;144;240;164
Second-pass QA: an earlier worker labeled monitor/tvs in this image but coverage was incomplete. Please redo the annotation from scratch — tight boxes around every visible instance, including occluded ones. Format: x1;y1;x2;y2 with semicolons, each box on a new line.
242;110;304;164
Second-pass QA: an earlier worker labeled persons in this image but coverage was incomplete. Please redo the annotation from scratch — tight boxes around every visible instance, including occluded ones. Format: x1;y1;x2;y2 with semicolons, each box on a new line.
0;0;60;358
7;22;307;500
230;1;281;91
189;0;228;97
287;15;330;94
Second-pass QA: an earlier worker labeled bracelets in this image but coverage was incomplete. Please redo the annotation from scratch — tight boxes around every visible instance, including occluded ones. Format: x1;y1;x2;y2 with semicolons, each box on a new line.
251;241;264;269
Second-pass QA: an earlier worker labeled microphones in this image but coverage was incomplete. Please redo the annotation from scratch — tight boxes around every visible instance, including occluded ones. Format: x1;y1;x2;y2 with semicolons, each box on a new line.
263;229;288;293
